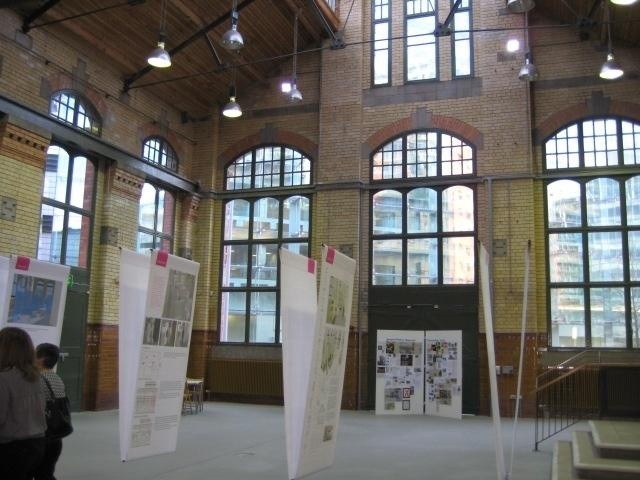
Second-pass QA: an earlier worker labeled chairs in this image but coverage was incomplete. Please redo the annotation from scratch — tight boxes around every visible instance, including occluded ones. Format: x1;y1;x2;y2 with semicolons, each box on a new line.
180;383;198;416
187;377;211;415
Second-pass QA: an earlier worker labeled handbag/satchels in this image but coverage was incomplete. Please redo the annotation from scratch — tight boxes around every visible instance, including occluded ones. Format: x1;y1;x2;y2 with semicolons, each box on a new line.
45;397;72;438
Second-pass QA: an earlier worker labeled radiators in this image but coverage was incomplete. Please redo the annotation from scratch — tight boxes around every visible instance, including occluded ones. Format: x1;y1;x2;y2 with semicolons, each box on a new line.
546;363;640;409
204;358;283;400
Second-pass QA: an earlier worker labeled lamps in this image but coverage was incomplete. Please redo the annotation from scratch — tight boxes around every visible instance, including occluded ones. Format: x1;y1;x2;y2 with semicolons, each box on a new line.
147;0;171;70
600;1;624;80
289;12;302;102
518;4;539;81
222;53;242;118
219;0;244;51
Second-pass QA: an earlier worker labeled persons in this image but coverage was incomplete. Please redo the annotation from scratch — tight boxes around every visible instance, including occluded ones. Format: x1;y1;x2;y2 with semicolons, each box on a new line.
0;327;48;480
34;342;66;480
